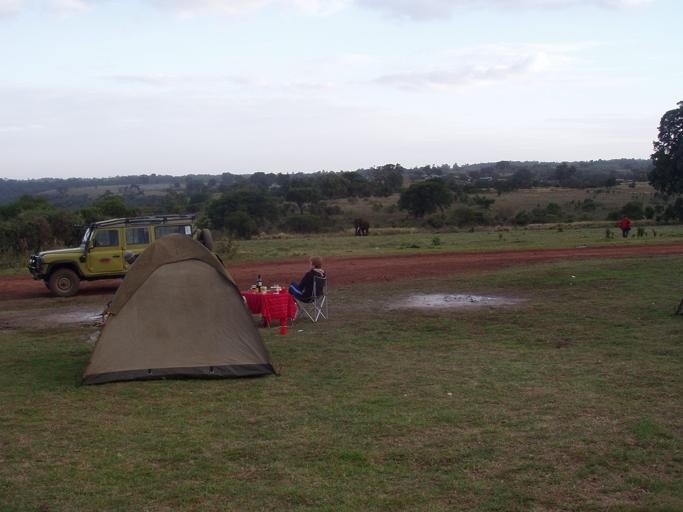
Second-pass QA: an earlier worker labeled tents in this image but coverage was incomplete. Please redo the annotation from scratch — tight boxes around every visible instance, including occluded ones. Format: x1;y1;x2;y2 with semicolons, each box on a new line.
80;233;279;386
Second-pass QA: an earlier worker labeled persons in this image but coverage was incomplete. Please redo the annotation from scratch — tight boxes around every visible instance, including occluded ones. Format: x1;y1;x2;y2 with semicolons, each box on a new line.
619;214;632;239
289;256;327;303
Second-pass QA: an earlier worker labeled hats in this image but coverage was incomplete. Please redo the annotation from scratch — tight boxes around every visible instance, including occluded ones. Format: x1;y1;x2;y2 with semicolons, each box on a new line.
125;252;135;260
311;256;323;266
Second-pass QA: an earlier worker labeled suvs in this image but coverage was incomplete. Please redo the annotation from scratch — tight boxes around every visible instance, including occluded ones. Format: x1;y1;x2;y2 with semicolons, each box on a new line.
28;215;216;297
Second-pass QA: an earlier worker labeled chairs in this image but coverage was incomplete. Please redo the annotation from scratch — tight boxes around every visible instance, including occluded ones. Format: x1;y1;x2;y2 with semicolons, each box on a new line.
293;275;328;327
